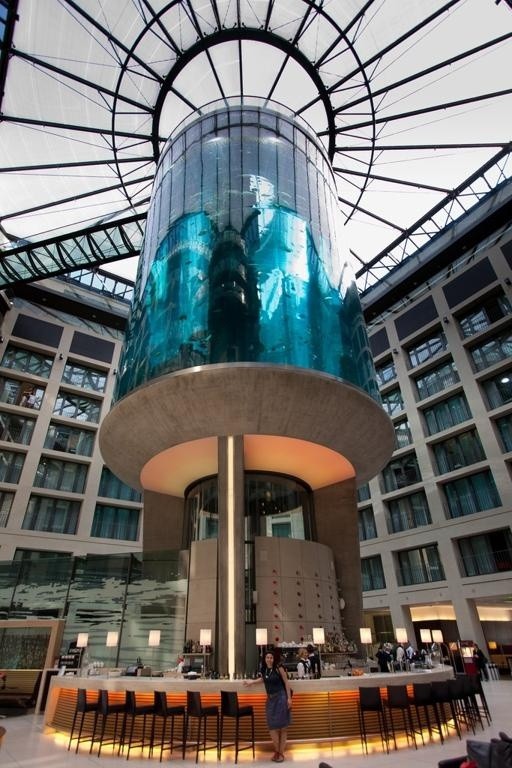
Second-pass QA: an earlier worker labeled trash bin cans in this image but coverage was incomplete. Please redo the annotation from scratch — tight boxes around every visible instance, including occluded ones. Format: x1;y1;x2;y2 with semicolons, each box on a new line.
484;663;500;680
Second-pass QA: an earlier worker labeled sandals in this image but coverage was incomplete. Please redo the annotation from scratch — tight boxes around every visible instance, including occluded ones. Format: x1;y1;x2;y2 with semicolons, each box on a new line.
272;752;284;762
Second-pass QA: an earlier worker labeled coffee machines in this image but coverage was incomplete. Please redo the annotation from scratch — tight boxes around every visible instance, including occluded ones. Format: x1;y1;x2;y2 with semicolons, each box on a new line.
274;647;319;671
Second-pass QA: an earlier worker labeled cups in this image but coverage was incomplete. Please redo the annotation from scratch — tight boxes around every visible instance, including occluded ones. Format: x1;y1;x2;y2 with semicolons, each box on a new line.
277;639;314;646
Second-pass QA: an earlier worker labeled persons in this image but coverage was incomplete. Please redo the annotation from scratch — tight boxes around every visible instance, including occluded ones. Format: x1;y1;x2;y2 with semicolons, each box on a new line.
374;640;489;681
242;645;321;762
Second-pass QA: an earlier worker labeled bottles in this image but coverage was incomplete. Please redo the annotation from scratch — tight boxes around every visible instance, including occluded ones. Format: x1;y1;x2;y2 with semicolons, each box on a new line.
387;652;433;672
183;639;213;653
87;660;105;675
210;671;263;679
286;662;319;679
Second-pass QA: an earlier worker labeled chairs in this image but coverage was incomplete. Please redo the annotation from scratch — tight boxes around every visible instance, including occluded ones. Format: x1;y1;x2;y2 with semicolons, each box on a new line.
68;686;257;764
356;673;491;754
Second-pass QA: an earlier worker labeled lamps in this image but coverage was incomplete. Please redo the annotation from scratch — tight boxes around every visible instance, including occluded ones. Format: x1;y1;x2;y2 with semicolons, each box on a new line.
77;633;88;678
199;628;211;680
256;628;268;678
360;628;372;675
148;630;161;680
419;629;431;670
312;628;325;678
395;627;408;673
432;629;443;670
106;631;119;678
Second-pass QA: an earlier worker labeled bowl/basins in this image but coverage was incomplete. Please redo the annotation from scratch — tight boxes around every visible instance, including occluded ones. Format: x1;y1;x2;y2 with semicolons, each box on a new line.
152;671;163;676
108;669;122;676
